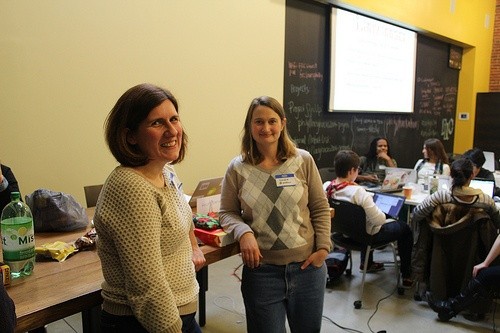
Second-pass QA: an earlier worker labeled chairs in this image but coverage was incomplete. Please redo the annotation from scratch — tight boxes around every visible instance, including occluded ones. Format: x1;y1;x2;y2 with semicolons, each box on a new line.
329;199;404;309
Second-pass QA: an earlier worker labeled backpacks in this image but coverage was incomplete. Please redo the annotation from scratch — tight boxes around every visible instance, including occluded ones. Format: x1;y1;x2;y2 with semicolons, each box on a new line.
325;244;349;277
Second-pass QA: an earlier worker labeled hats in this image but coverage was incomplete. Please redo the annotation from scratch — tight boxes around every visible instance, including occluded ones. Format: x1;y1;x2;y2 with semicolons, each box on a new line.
463;148;486;166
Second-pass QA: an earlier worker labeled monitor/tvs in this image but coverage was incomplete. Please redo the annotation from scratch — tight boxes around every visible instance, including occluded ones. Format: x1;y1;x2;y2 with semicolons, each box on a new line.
385;167;417;184
469;177;495;200
482;151;495;173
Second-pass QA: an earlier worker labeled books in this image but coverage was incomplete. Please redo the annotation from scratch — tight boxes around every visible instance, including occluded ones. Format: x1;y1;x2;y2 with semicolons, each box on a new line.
192;227;235;248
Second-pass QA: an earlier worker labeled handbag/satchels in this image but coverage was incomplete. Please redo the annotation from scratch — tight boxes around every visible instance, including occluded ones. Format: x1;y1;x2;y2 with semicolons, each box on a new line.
194;228;238;248
25;189;88;233
402;278;417;288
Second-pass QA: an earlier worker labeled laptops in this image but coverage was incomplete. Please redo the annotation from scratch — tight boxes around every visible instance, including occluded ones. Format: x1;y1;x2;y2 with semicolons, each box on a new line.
373;192;406;221
184;176;224;208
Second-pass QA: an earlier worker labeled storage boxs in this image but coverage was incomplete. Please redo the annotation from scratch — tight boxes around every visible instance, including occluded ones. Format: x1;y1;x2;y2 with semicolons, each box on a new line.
191;226;237;247
0;263;10;288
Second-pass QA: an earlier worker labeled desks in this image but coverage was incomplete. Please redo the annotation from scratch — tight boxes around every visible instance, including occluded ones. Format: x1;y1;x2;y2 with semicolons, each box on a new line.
360;185;500;246
0;207;241;333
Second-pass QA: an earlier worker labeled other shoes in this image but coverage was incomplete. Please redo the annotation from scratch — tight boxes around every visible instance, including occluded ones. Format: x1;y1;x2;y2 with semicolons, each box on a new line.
360;262;386;272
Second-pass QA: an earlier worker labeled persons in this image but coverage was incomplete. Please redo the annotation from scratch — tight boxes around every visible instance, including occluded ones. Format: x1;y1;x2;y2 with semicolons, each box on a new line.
411;158;499;301
0;164;23;221
0;282;17;333
399;137;451;223
356;138;397;252
93;83;206;333
464;149;498;197
220;96;333;333
466;233;500;322
319;149;412;289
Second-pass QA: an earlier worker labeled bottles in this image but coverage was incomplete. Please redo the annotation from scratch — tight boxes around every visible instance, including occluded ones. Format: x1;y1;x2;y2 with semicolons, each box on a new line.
430;176;439;195
424;171;429;193
0;192;36;281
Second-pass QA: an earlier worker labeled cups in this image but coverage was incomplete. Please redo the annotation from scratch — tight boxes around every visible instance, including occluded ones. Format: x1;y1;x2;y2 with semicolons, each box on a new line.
403;187;413;200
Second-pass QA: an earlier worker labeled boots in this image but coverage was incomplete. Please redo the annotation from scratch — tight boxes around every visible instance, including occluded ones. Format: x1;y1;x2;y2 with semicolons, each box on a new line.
428;278;482;322
